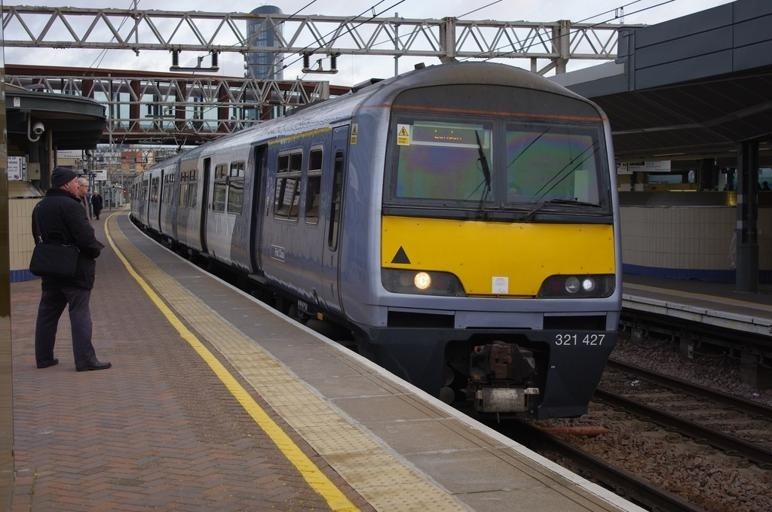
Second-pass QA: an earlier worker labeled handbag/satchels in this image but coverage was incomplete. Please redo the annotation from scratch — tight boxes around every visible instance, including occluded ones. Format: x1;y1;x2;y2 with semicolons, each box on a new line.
30;244;79;279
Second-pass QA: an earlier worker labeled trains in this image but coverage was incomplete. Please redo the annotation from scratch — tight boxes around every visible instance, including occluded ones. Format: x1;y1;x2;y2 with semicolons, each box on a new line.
128;60;623;426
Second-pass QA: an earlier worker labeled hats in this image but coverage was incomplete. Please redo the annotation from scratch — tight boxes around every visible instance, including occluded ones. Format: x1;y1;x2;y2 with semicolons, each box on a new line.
51;168;76;186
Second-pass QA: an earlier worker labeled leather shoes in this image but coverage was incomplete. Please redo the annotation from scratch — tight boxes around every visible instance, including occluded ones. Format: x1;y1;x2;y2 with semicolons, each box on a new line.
77;360;111;371
37;359;58;368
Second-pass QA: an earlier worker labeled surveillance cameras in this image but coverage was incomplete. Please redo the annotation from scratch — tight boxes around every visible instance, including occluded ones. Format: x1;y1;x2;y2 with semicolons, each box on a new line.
33;122;45;135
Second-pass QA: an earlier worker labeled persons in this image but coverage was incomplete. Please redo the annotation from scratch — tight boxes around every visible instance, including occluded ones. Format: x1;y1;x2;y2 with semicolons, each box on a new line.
32;167;111;371
91;190;103;220
77;178;92;224
